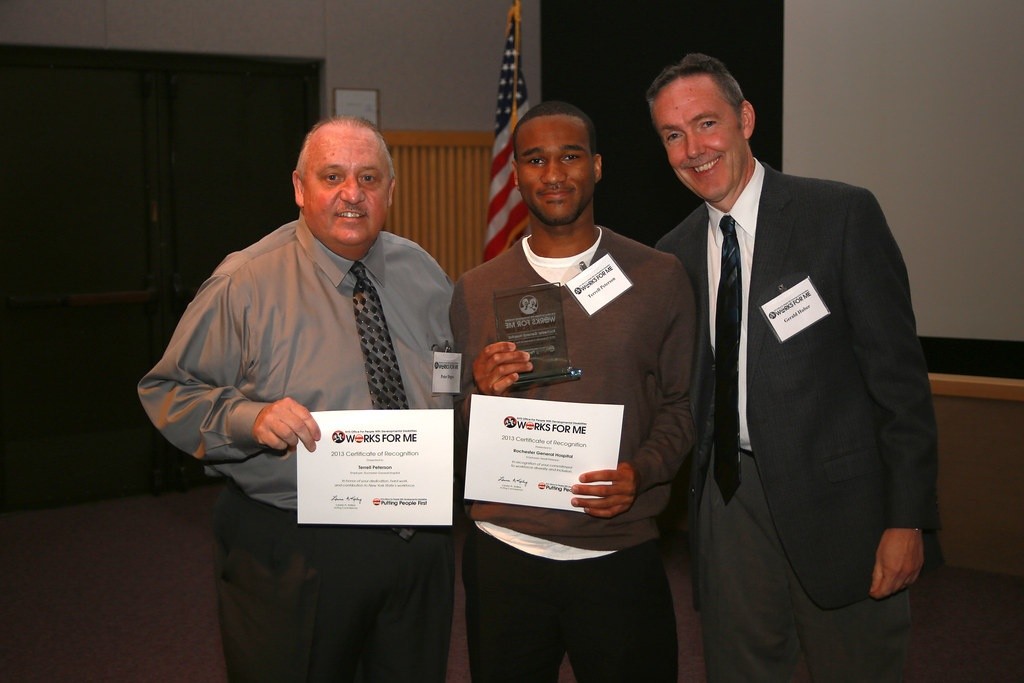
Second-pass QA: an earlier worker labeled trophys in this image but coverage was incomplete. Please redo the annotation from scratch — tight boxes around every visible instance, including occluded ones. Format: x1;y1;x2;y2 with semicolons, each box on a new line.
492;281;582;391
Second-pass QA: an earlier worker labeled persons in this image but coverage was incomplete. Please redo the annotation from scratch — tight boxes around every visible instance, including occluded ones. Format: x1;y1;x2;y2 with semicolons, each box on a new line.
451;102;701;683
648;53;940;683
136;112;461;682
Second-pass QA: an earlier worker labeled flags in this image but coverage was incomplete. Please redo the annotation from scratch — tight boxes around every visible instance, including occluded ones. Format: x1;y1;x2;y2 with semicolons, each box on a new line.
479;0;534;265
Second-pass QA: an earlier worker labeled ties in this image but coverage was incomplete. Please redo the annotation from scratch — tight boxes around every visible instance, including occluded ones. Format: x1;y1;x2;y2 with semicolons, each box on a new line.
351;260;420;542
713;215;743;509
